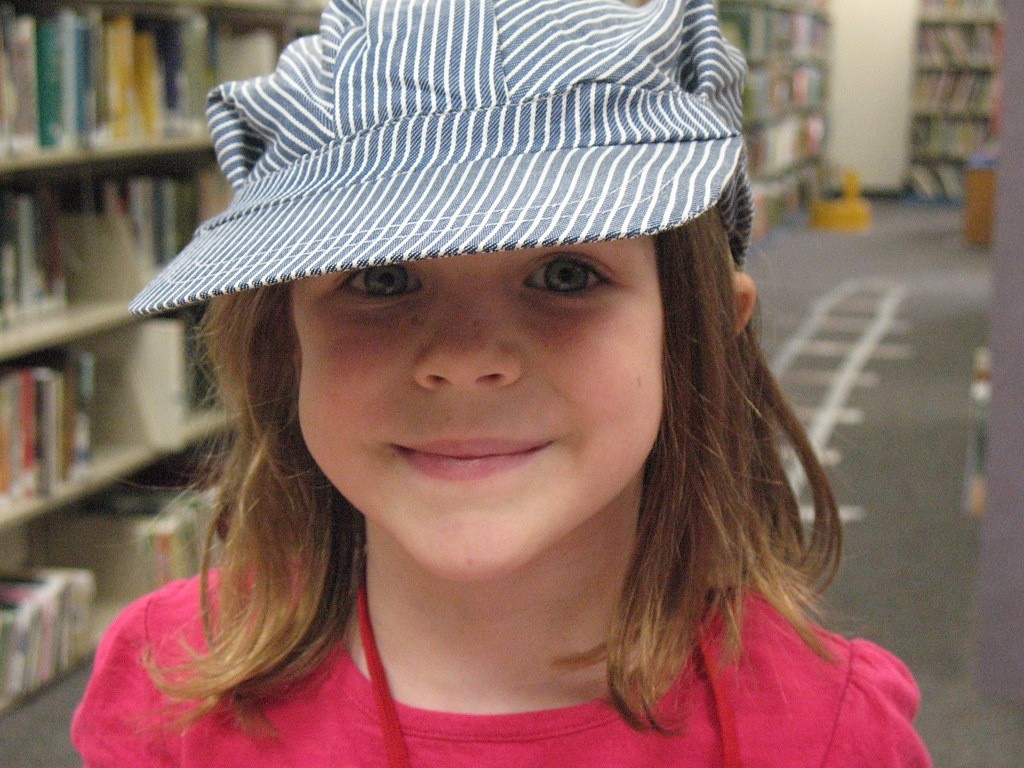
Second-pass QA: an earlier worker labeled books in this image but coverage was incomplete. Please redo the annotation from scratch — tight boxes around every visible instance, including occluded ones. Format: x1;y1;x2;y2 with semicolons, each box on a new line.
717;2;828;233
2;445;237;708
2;342;95;511
913;18;999;201
1;9;283;157
0;161;207;322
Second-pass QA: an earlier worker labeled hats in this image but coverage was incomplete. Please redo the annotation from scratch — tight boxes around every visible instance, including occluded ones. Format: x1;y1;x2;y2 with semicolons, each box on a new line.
126;0;752;328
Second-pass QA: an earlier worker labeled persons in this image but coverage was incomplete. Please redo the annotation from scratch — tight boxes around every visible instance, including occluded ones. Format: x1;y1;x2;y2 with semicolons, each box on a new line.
62;0;935;766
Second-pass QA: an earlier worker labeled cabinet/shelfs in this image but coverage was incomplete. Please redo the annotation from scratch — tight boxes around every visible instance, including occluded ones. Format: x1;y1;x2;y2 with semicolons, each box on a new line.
0;0;333;723
713;0;833;237
897;0;1006;209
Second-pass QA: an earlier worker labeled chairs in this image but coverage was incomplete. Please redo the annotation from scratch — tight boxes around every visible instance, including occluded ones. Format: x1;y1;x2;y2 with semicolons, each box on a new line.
810;163;871;233
